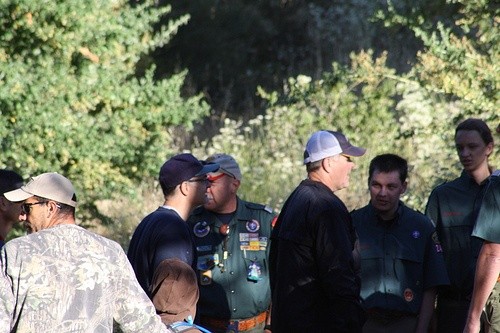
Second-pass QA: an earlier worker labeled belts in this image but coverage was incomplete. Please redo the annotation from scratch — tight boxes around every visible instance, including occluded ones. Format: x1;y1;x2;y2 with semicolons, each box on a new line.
228;311;269;331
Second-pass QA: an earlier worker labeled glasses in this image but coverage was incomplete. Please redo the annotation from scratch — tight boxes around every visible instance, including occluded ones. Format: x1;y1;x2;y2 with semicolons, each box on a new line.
187;174;209;185
21;200;48;216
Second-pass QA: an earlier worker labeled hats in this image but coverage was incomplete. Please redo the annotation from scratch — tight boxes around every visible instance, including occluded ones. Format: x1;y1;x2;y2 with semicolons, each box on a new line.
158;152;220;196
301;130;367;165
4;171;78;208
205;153;242;181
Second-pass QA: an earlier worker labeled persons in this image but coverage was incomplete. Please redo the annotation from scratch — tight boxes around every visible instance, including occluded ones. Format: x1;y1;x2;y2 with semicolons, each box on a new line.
128;153;219;295
460;174;500;333
348;154;450;333
151;259;200;333
423;118;498;333
269;129;367;333
0;173;175;333
1;169;26;243
190;153;279;333
